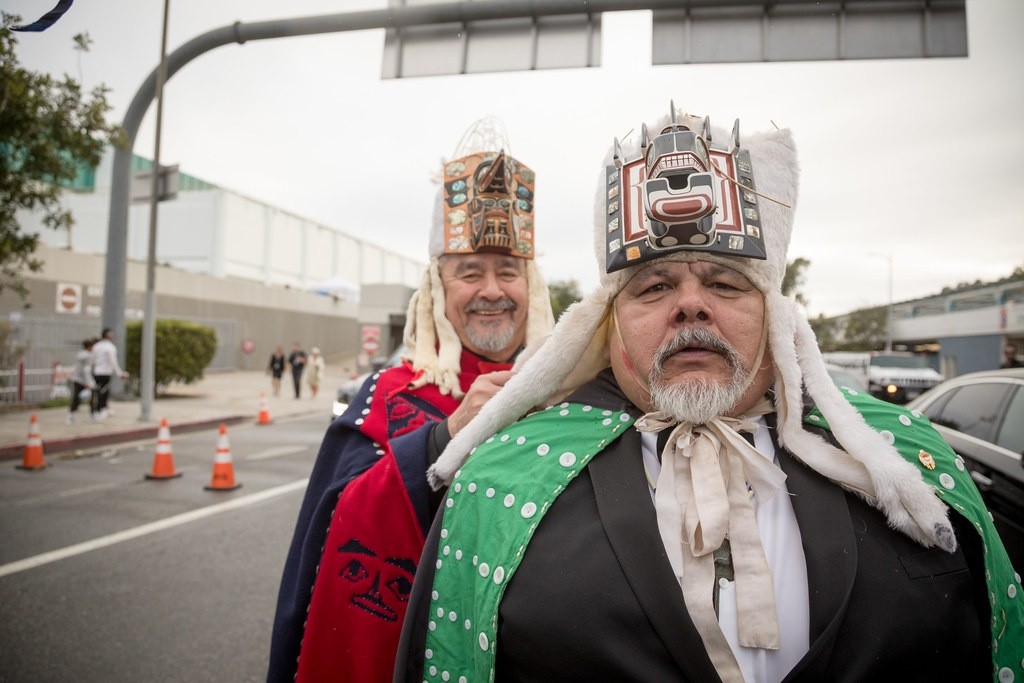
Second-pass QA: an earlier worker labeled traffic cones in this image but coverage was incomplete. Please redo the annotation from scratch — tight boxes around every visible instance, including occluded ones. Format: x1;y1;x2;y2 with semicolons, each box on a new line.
256;391;275;426
142;417;182;480
13;414;52;470
204;423;242;491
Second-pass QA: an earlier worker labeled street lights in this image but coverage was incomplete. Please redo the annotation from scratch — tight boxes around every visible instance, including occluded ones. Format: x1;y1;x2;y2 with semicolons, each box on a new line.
856;249;892;351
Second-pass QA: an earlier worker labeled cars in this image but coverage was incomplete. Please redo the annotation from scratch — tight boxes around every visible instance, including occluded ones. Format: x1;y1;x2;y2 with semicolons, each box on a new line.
333;367;378;422
904;367;1024;589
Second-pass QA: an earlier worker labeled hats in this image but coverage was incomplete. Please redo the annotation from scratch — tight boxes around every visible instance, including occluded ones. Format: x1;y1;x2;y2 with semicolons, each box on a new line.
429;98;959;555
401;150;556;399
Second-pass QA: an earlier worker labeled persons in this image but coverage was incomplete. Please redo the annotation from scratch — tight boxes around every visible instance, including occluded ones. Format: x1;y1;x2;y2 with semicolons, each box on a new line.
66;327;124;424
266;151;556;683
307;347;325;400
1000;343;1024;369
266;346;285;396
288;343;306;399
423;101;1024;683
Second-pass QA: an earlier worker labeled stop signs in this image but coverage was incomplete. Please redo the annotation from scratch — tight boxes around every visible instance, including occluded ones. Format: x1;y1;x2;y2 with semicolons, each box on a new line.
56;283;81;313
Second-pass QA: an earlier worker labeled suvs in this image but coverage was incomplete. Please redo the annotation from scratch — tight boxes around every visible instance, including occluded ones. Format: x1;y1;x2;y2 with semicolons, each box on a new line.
864;352;944;401
